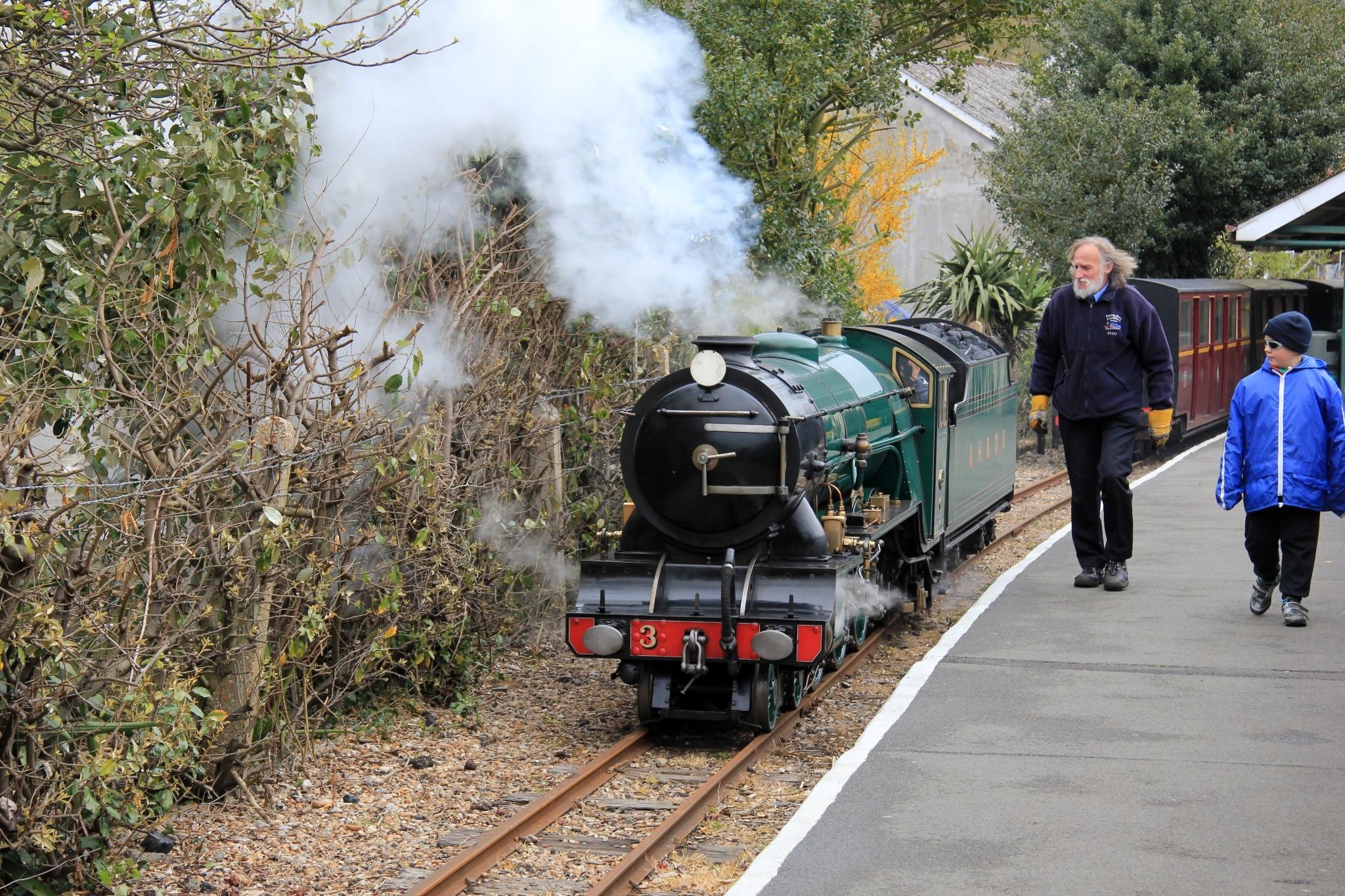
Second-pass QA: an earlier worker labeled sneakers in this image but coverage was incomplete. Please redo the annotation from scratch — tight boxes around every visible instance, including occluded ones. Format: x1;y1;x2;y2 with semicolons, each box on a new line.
1074;566;1104;587
1281;597;1309;626
1250;570;1281;614
1103;560;1129;590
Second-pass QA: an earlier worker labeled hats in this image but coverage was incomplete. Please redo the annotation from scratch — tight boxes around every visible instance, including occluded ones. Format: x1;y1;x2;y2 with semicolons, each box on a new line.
1263;311;1313;353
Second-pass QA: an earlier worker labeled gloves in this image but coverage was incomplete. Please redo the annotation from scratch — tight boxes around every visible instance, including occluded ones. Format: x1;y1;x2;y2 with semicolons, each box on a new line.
1029;395;1049;435
1147;408;1173;452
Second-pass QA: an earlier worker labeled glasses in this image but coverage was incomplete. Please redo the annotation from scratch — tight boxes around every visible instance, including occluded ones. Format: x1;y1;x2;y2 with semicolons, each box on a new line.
1263;338;1283;349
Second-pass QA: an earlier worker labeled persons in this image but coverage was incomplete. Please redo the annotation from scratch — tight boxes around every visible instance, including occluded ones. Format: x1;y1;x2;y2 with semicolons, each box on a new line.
1215;311;1345;628
1030;234;1175;591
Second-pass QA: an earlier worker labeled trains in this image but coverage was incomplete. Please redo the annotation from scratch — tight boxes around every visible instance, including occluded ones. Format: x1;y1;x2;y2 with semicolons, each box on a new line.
569;318;1020;733
1126;277;1345;461
